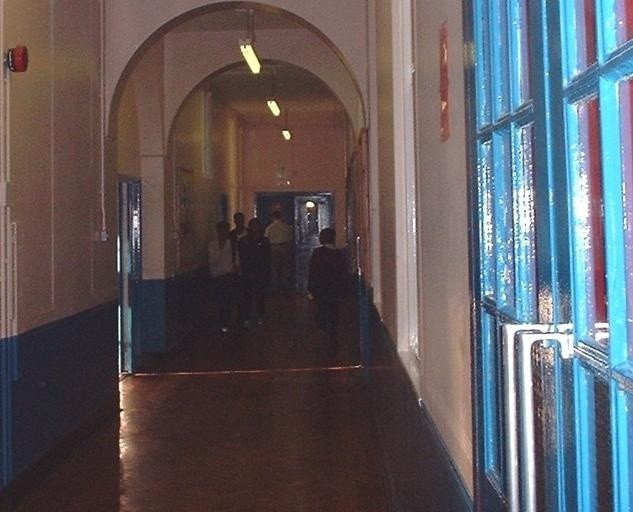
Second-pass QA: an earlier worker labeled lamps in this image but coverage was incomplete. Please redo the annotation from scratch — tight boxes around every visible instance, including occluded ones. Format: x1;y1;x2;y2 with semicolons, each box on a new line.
266;81;281;118
280;108;293;141
237;7;264;76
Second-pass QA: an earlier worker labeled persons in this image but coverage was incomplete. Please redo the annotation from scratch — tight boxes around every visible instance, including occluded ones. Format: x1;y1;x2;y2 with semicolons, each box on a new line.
307;228;352;358
207;210;293;335
